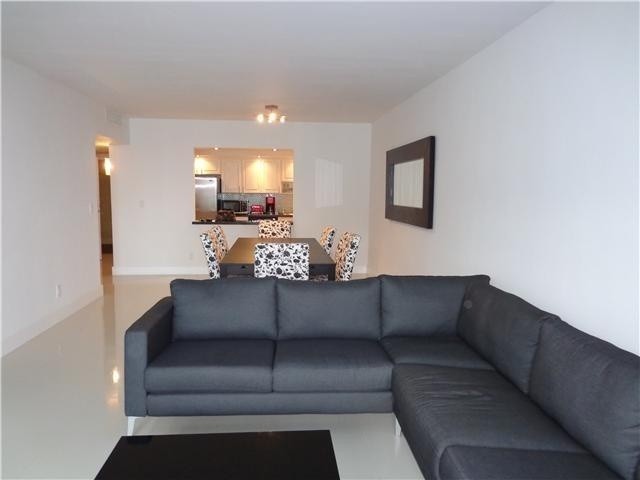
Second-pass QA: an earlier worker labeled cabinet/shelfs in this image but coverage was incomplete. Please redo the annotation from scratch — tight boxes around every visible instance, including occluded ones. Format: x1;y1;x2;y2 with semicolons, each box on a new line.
195;148;294;194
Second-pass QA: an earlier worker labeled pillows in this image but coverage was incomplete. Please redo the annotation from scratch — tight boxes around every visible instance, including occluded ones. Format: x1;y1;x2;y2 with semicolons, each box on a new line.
378;274;491;338
170;276;278;341
457;277;554;394
528;313;640;480
276;278;383;342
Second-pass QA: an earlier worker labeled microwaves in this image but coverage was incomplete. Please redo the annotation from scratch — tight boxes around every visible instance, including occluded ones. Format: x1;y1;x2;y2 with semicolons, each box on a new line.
219;199;249;214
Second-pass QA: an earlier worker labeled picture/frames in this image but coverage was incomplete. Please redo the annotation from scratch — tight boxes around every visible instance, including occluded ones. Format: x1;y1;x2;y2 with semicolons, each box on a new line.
385;136;435;229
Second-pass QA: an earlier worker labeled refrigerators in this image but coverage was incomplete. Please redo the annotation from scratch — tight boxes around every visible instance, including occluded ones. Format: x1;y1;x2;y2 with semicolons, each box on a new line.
195;177;220;219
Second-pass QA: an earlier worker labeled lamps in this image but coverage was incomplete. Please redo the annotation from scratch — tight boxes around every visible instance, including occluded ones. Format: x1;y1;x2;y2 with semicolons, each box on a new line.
257;106;287;123
105;157;110;175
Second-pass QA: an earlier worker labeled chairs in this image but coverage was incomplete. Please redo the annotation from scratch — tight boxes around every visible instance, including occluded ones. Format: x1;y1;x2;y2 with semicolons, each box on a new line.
258;219;291;239
319;226;336;255
309;232;361;282
211;226;229;255
254;242;310;282
200;230;247;279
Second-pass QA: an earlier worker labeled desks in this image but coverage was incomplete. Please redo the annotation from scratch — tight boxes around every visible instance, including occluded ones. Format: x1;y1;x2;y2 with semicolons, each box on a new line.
219;237;336;281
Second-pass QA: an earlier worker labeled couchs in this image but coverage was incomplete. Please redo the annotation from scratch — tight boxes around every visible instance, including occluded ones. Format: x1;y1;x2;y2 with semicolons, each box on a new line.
125;275;640;480
